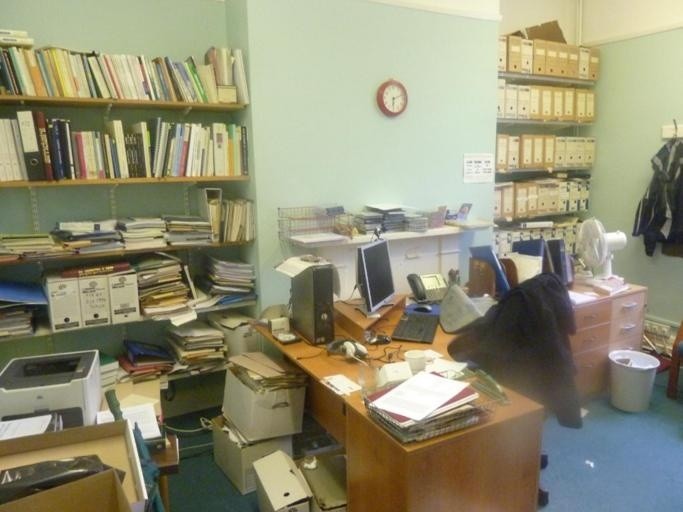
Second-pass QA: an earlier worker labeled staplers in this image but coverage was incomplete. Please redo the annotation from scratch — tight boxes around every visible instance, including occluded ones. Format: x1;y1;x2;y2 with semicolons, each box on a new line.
473;369;505;402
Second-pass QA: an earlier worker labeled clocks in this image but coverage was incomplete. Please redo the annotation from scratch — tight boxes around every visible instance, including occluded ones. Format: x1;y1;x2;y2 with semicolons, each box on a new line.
377;79;408;116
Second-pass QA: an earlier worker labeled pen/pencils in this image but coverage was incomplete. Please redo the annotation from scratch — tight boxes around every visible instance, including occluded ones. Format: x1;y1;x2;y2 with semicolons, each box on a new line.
448;268;460;283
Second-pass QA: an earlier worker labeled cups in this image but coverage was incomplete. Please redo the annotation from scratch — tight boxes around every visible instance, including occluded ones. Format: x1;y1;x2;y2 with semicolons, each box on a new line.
214;48;233;87
403;349;426;375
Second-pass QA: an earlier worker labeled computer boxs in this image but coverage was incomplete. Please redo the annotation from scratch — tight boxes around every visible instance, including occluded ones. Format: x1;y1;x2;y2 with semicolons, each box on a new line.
290;264;335;345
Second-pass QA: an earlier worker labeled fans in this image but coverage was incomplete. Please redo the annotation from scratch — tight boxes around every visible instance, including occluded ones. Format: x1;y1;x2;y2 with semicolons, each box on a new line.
575;218;627;280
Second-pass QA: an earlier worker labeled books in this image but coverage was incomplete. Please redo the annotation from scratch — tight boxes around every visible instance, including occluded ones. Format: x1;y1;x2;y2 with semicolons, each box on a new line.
1;188;255;386
0;29;249;106
1;109;248;183
364;373;479;444
355;204;428;233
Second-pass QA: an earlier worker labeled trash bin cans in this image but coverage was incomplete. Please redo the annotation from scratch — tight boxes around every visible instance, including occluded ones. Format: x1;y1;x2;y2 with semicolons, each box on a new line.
608;350;661;413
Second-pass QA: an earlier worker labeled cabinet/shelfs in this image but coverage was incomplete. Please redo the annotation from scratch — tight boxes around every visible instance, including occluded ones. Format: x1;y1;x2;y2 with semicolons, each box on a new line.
565;285;646;405
2;95;258;420
494;75;599;257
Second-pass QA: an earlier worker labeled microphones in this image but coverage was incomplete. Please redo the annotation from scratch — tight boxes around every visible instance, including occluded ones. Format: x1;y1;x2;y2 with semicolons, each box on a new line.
346;349;368;367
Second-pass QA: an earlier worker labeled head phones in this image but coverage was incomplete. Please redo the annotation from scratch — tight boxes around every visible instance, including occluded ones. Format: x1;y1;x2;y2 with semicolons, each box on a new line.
327;338;369;357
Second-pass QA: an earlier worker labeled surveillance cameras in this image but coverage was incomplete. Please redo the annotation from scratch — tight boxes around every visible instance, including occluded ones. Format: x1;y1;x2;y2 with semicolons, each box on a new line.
374;226;383;241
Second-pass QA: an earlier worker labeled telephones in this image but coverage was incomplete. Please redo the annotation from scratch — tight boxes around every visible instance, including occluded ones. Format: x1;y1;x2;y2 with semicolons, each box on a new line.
407;273;449;304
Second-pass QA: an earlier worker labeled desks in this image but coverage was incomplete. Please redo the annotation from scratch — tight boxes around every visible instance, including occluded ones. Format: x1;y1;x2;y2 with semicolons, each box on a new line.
251;296;545;512
291;222;492;301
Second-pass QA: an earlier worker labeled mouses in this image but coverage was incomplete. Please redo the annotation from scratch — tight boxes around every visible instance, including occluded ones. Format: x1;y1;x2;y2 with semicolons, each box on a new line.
368;335;391;344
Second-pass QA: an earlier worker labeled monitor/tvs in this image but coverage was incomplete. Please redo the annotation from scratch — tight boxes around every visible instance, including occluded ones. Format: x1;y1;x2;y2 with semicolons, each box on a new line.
355;238;394;318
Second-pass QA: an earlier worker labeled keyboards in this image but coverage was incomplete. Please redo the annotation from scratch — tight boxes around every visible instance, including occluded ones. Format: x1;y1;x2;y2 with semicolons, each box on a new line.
391;312;439;344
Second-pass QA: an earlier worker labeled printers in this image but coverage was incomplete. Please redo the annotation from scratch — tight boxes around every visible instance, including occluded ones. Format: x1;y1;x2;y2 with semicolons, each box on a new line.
0;348;104;431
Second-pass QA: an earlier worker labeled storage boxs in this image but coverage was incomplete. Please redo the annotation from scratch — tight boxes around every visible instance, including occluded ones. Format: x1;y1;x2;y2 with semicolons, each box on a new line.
1;420;148;511
221;369;307;441
300;448;348;510
210;416;295;496
251;450;314;511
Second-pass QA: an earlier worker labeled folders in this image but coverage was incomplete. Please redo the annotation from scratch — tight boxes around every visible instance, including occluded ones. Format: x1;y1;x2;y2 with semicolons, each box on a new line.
496;133;596;172
196;186;222;243
496;79;595;122
494;177;590;219
497;34;600;81
16;110;46;181
117;340;176;375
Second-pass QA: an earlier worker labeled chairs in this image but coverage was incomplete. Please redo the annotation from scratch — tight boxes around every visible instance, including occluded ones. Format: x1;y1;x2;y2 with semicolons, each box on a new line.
667;321;683;399
